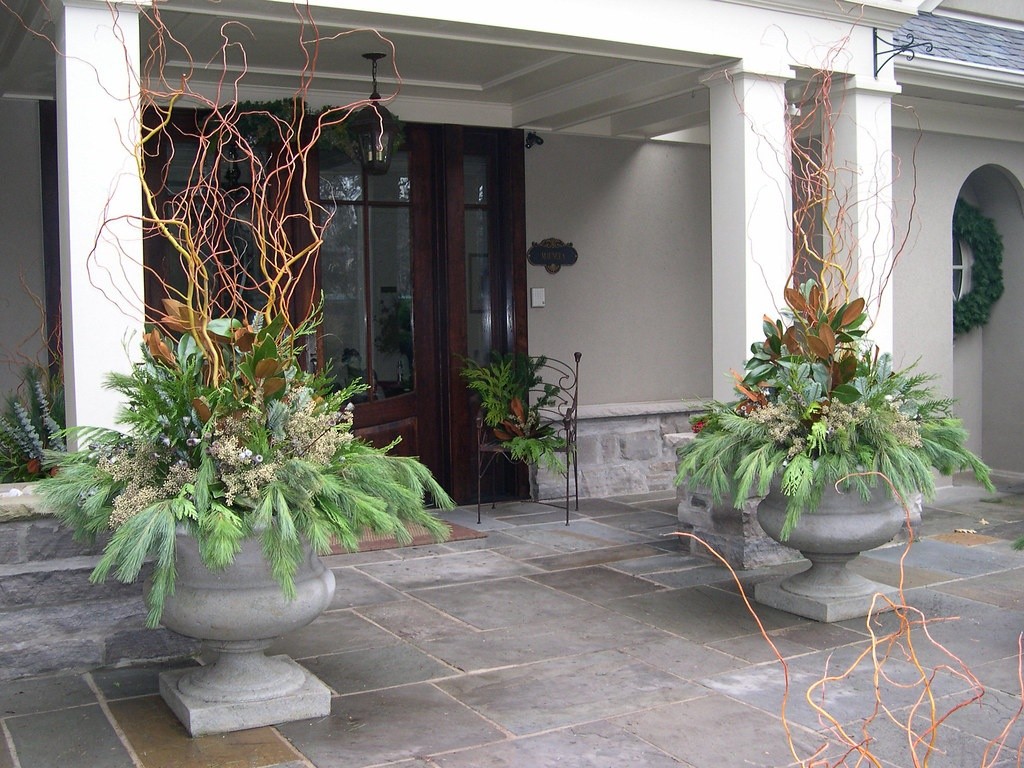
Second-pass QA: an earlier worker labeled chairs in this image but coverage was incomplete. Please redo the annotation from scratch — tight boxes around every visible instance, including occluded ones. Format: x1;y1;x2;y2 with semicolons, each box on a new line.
474;351;583;527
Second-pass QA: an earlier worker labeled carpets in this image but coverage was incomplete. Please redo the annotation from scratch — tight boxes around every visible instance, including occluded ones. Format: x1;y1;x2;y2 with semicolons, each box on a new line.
317;515;487;557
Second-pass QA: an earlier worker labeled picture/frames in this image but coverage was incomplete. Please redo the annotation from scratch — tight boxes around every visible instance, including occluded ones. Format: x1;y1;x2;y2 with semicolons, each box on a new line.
468;252;490;314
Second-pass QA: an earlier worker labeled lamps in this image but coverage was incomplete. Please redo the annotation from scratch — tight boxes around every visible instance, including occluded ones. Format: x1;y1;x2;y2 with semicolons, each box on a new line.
348;52;400;176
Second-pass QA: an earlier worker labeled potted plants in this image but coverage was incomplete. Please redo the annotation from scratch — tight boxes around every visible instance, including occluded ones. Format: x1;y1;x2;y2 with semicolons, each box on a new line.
0;0;462;741
667;0;998;626
